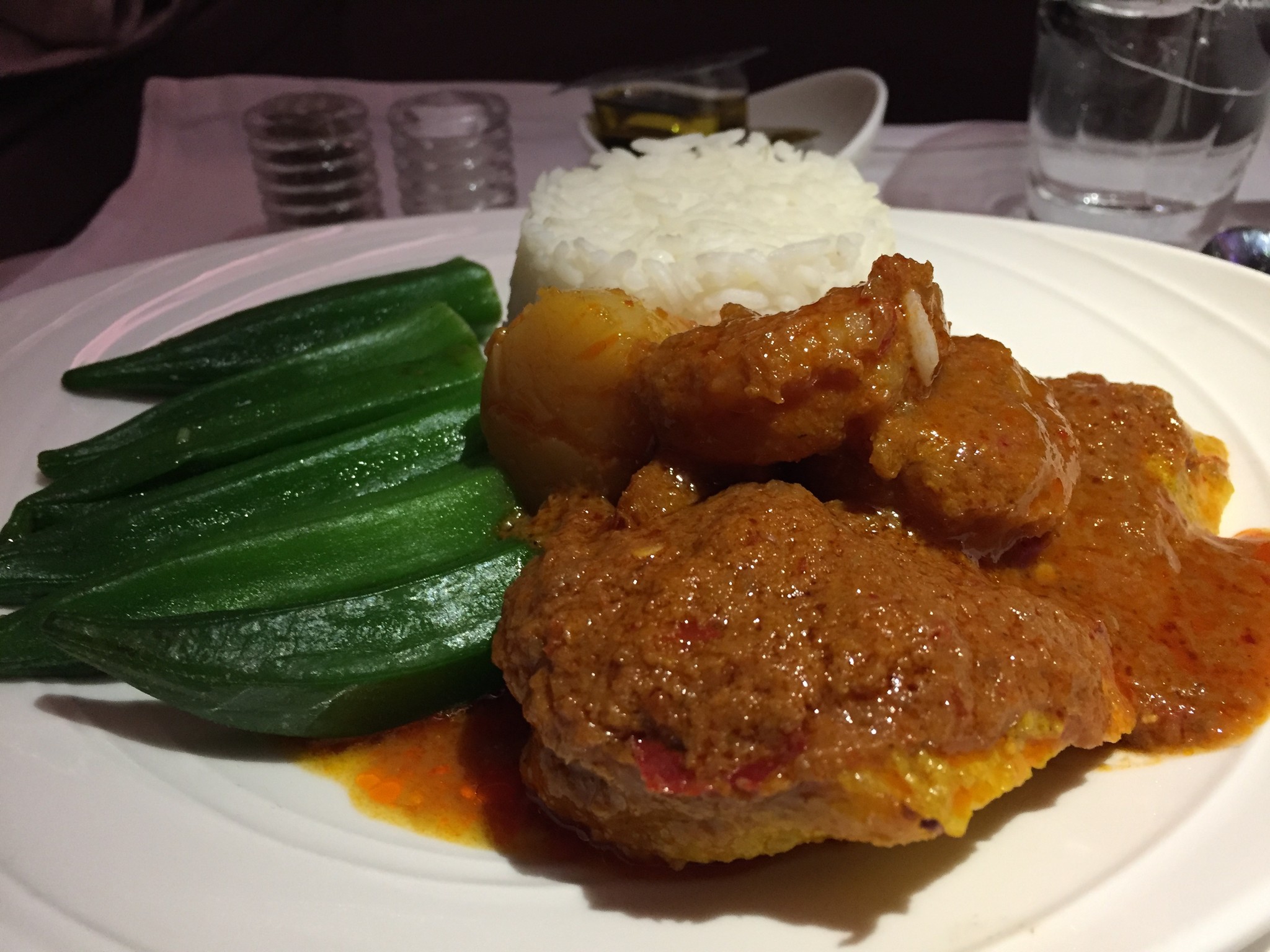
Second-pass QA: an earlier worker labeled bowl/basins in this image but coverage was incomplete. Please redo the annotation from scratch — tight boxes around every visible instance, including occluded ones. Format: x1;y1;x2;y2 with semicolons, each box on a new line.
576;68;890;173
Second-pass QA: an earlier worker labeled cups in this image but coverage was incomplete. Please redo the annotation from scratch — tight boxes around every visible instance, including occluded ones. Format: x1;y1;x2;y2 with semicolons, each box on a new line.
1021;0;1270;259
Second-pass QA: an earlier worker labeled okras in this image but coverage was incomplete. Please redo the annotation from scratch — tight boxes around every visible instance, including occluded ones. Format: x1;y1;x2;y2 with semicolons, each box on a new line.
0;256;536;741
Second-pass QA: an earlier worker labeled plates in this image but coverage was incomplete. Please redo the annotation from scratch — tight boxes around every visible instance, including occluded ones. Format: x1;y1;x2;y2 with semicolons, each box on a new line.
0;202;1268;952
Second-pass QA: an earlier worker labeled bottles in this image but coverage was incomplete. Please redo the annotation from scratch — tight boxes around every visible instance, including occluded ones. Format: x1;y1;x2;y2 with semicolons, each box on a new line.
383;89;521;215
240;90;389;230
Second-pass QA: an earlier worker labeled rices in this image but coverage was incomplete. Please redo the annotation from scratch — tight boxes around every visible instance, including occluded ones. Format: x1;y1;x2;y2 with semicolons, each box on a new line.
503;127;895;327
